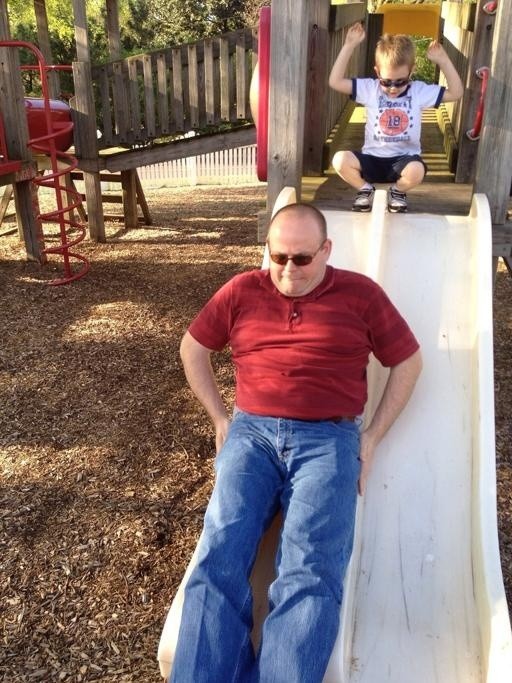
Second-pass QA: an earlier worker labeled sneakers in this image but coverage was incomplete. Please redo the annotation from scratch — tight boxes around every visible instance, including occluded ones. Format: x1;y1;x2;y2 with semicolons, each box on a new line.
352;185;376;213
387;186;408;212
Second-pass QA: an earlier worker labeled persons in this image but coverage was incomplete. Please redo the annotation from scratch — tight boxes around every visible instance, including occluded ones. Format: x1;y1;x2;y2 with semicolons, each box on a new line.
168;202;424;683
328;22;464;213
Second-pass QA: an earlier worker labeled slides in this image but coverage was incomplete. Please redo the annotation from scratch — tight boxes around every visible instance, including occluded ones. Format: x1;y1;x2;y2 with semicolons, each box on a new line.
158;186;510;682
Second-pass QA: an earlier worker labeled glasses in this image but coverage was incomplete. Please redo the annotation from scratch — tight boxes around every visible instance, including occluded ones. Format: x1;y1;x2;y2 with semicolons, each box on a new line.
378;71;411;88
267;239;327;267
